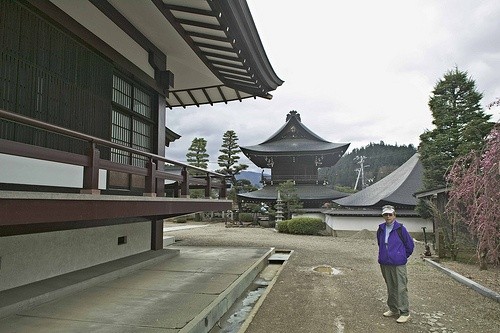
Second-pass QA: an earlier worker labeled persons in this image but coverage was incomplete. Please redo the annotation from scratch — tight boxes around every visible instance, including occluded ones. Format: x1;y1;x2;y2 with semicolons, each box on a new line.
377;205;414;323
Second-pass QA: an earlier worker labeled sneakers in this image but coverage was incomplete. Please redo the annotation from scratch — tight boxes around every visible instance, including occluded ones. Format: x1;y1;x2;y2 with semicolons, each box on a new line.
383;310;400;317
396;315;410;322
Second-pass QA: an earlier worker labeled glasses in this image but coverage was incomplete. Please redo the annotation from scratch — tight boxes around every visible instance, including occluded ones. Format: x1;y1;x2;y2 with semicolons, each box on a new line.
384;213;393;216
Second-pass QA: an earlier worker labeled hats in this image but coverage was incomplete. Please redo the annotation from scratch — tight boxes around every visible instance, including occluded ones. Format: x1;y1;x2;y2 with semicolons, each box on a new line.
382;205;395;215
